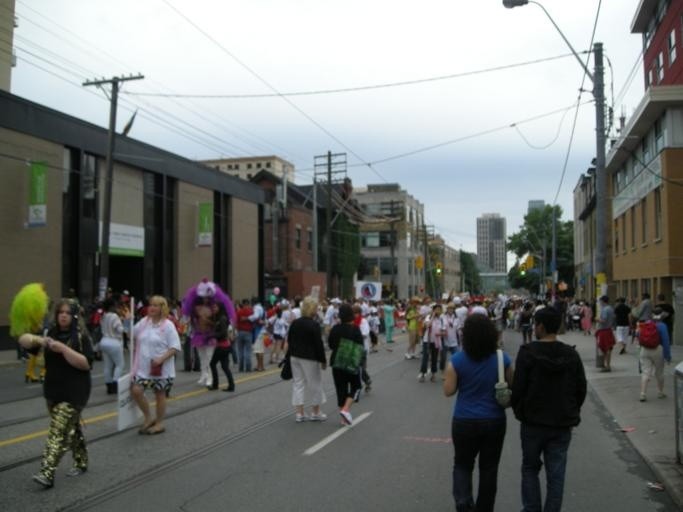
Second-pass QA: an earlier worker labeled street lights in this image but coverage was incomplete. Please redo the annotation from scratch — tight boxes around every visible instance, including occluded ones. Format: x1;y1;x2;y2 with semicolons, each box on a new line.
502;0;611;367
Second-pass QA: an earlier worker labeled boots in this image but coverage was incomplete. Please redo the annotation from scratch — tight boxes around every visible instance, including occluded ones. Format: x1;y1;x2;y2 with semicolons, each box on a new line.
106;381;118;394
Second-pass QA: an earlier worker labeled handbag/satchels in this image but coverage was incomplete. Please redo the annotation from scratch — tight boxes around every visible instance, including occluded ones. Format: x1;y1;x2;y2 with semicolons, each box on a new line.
333;338;364;374
280;352;292;379
495;382;512;408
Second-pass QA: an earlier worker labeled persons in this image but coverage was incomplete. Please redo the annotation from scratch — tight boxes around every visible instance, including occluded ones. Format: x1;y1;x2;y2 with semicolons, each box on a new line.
18;298;96;487
595;293;676;372
492;292;593;343
328;304;364;427
88;287;489;392
128;294;181;435
286;296;328;423
514;305;586;510
636;307;672;403
25;317;47;383
445;314;513;511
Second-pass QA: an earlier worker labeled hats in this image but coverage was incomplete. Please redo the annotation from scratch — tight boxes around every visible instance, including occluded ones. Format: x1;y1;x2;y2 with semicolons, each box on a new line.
282;299;289;308
454;296;461;303
411;295;423;302
447;303;455;310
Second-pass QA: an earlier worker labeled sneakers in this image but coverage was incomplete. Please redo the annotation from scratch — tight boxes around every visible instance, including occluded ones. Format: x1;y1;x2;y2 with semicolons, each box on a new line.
179;361;267;391
657;391;667;399
146;424;166;434
601;368;610;372
640;393;647;401
620;349;626;354
405;352;447;382
32;473;54;488
66;467;87;477
295;410;352;425
138;421;155;434
366;381;372;392
